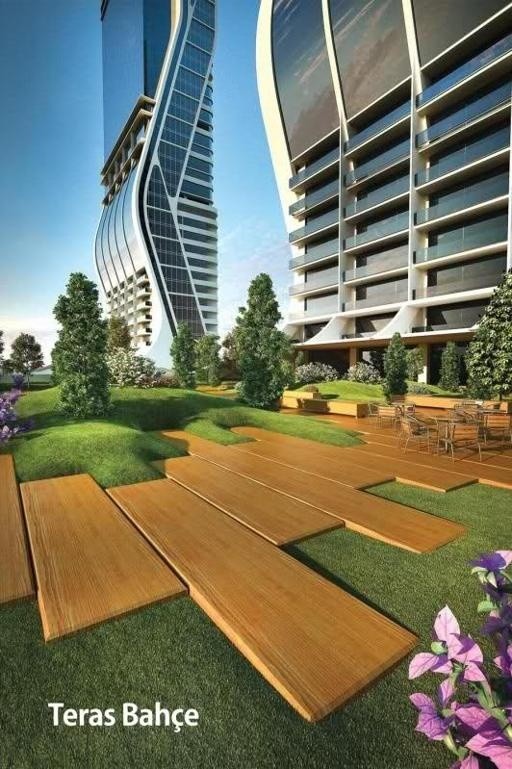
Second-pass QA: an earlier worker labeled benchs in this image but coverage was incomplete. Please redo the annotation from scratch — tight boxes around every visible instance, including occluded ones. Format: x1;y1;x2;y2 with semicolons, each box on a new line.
397;414;430;454
282;391;322;399
281;397;302;409
302;399;368;419
437;423;482;465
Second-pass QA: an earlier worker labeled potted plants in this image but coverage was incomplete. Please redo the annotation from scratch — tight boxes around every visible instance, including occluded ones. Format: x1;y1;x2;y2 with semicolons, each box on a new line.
382;333;409;403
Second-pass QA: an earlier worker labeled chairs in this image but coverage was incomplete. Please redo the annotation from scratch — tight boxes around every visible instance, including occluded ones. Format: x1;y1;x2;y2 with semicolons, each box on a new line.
368;400;512;445
